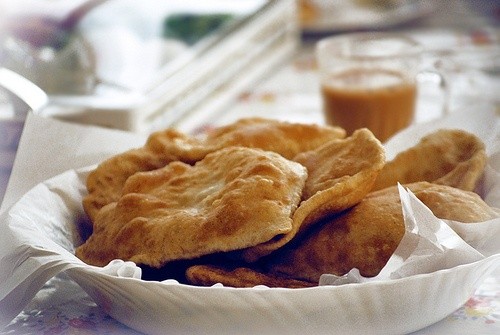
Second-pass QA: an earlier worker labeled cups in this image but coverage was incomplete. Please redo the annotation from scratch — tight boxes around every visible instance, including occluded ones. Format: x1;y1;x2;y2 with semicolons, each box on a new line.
314;31;423;145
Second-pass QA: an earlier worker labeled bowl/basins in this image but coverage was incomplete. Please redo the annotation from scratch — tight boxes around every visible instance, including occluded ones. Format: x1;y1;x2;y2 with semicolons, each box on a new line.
4;149;499;335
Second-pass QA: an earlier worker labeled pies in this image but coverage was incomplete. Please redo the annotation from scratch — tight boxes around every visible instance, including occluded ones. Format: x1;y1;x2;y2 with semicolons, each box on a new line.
80;122;498;287
